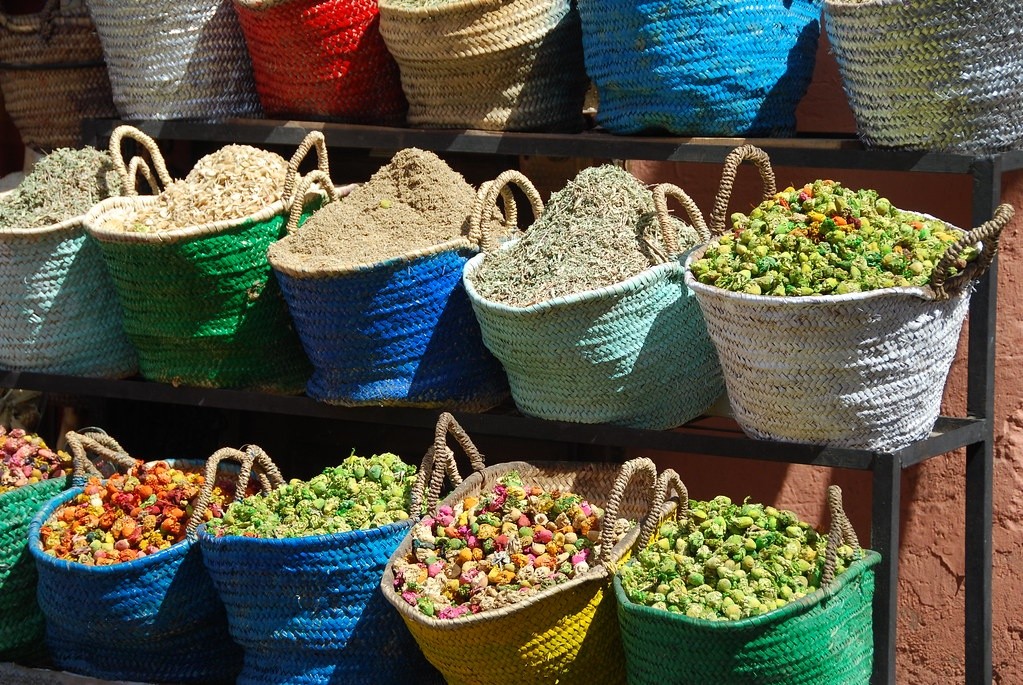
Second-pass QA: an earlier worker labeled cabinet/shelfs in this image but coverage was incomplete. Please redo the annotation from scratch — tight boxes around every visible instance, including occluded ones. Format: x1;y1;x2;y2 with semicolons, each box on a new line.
0;93;1023;685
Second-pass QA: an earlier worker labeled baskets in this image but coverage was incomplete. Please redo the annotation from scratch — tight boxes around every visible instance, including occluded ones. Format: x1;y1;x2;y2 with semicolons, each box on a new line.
0;125;1013;453
1;426;881;685
0;0;1023;153
